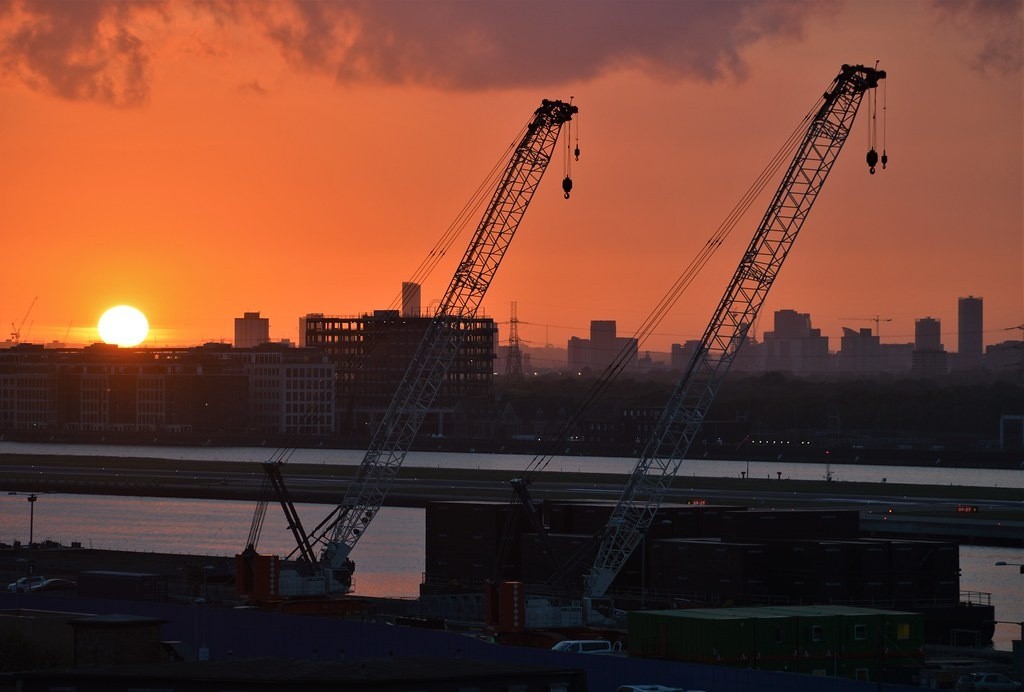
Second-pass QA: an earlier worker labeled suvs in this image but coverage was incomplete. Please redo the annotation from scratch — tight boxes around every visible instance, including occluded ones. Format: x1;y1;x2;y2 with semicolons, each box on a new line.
7;576;46;593
957;672;1024;692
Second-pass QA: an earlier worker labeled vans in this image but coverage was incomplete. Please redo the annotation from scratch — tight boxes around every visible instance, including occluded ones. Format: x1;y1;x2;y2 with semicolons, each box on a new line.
552;639;611;654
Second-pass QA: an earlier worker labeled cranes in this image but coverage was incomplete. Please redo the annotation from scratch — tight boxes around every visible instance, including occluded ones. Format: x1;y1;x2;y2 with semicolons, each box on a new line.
228;97;580;616
483;63;888;647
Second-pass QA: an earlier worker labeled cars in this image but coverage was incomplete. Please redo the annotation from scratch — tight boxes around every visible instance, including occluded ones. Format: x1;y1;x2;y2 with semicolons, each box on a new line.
26;578;78;592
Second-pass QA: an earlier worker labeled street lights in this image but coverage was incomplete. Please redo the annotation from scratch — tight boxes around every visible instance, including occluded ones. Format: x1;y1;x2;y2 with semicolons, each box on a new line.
203;565;215;649
8;491;56;545
983;619;1024;671
